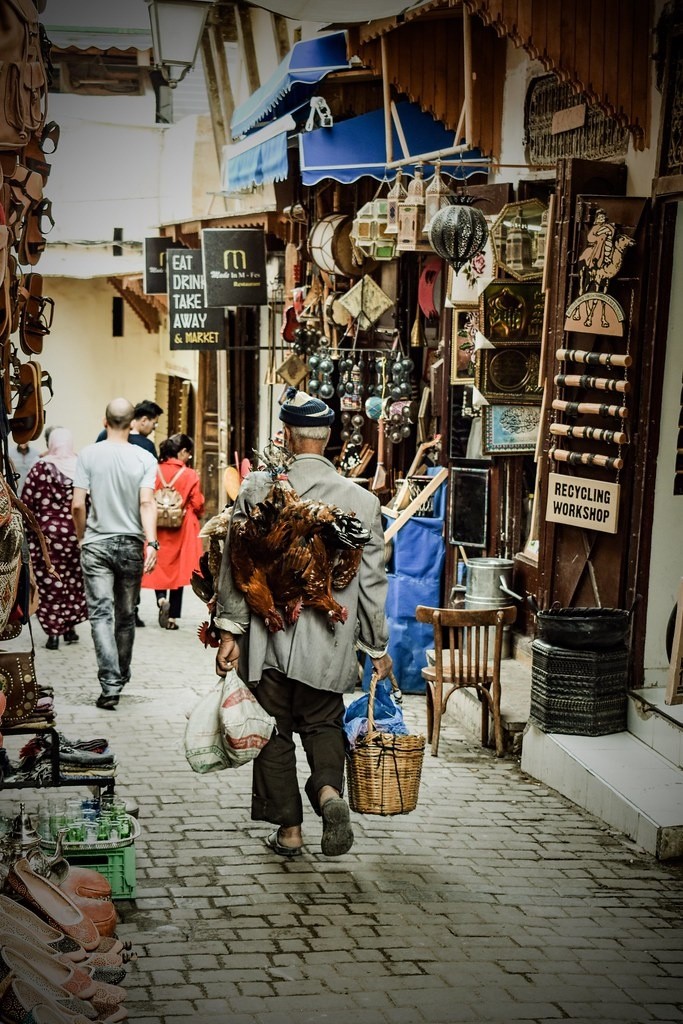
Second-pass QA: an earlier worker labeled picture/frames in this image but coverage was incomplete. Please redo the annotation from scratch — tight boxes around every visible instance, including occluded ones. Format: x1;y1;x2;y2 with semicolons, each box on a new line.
483;399;542;456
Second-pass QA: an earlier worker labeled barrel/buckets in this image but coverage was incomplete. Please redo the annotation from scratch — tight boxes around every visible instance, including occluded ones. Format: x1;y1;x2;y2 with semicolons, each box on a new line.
463;557;516;663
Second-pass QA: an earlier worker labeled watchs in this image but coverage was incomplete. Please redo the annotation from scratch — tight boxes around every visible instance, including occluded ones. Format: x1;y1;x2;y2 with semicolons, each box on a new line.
146;539;160;551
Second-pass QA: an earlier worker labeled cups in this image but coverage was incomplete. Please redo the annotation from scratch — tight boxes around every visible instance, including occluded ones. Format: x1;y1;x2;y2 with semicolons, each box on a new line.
36;794;133;843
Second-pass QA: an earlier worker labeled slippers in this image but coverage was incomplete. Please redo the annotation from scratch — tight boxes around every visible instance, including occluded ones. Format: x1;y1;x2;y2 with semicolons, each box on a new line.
262;828;303;857
321;798;354;857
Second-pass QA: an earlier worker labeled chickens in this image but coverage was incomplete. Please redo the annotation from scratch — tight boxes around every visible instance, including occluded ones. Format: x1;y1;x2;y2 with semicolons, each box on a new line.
190;439;373;650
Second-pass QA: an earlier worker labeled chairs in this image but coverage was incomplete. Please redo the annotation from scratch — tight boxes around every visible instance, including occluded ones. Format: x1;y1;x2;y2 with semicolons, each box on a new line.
416;606;517;757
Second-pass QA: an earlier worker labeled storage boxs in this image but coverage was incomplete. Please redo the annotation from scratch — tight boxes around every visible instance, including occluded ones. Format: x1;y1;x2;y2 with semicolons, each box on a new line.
36;812;143;900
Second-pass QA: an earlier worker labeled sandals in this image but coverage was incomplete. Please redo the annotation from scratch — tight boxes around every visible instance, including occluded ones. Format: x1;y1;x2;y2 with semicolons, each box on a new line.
158;601;179;629
0;121;61;448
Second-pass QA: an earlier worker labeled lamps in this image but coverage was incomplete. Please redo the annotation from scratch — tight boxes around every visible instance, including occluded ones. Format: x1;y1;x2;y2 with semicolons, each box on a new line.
429;153;489;278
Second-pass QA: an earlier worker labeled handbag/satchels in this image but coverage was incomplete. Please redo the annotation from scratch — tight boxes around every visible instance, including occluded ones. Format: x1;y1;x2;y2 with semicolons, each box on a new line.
0;454;46;642
0;651;38;725
181;662;277;774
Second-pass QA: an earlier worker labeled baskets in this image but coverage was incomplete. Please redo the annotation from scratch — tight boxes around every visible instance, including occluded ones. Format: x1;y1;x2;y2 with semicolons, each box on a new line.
537;606;630;651
346;666;426;818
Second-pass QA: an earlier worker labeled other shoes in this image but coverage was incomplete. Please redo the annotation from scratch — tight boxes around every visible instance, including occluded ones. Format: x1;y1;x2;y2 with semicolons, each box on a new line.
0;859;129;1024
63;630;79;641
46;635;59;650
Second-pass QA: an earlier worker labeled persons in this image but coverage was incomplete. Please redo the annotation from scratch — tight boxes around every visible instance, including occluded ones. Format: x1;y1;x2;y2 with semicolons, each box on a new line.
19;425;92;651
215;386;393;856
140;430;205;631
94;399;163;627
70;397;160;709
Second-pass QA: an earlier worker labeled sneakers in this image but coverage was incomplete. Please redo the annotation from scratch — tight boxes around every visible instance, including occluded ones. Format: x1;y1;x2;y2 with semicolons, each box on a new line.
96;693;120;708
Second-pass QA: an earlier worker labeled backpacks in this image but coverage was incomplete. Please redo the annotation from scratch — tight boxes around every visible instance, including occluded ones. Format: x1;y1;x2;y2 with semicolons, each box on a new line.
0;0;48;151
155;463;187;530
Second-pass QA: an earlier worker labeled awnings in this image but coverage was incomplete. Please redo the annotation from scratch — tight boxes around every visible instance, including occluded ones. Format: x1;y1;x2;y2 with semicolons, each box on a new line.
220;28;490;187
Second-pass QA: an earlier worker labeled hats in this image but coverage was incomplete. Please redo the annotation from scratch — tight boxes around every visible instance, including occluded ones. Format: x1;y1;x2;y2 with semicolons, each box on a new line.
279;385;335;427
